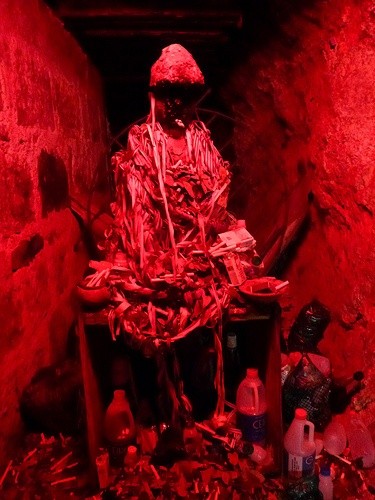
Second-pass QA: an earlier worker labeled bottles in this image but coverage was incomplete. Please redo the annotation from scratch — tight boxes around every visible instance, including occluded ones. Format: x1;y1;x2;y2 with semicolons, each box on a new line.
319;467;334;500
103;390;135;470
282;409;315;483
226;333;240;389
235;369;268;444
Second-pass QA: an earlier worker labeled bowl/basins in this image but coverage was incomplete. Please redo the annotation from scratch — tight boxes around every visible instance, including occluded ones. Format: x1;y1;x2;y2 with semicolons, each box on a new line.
238;277;290;302
